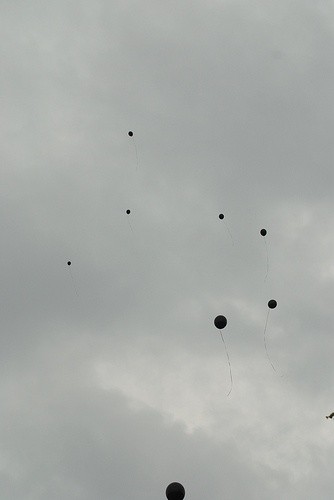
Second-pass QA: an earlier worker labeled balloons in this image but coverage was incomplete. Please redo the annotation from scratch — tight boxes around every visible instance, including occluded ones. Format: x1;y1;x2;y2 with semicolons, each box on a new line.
165;481;185;500
214;314;228;330
260;228;267;236
218;213;225;220
67;260;71;266
128;131;134;137
267;299;278;309
126;209;131;215
